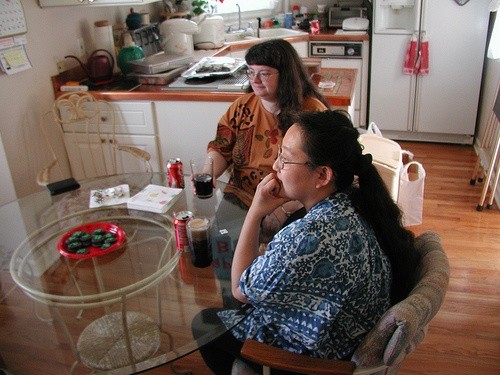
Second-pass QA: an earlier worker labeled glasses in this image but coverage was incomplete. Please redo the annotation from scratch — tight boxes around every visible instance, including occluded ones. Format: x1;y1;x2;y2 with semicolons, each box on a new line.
246;68;280;80
277;144;322;169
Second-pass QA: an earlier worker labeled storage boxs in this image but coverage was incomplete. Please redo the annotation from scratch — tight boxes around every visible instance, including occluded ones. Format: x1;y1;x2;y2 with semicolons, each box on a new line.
126;52;192;84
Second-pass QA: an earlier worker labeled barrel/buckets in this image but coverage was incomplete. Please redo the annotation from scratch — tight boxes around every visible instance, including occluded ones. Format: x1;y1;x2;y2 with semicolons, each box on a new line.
160;18;197;55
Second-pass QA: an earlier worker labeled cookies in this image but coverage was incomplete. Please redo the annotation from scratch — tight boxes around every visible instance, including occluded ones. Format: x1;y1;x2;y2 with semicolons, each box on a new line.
65;228;117;254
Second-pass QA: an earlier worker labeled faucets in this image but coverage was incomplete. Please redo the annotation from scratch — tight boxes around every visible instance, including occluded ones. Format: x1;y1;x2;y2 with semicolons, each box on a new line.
256;17;261;28
224;3;253;34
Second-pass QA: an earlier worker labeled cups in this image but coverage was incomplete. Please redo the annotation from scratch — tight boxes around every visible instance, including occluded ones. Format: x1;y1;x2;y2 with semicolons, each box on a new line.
191;156;215;200
141;13;151;25
186;215;212;268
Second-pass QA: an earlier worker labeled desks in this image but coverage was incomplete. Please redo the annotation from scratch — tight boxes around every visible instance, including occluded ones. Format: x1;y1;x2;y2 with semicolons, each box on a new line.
0;172;263;375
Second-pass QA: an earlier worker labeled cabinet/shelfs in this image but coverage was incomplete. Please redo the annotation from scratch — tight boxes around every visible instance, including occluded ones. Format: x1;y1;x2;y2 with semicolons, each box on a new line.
60;98;160;180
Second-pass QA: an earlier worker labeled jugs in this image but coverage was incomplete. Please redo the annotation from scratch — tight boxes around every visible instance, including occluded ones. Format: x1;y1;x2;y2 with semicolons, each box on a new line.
64;49;115;83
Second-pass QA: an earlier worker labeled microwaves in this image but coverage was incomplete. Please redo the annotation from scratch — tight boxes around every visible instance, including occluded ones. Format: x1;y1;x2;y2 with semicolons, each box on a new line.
328;7;367;29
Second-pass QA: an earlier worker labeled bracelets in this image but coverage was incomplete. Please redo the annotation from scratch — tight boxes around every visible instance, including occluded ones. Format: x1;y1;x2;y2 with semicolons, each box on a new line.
280;206;290;216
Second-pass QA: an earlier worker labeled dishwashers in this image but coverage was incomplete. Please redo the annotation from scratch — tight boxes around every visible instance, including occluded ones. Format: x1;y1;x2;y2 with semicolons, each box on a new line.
308;40;369;130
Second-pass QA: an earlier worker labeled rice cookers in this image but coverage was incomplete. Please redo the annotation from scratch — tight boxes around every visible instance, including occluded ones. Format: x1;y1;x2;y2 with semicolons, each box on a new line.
190;14;225;50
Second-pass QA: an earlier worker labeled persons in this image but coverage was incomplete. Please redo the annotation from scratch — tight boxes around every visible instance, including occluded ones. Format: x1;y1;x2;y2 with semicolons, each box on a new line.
192;109;419;375
192;39;330;309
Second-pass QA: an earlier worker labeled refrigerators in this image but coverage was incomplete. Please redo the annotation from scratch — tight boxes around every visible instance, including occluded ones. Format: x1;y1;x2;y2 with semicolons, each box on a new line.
369;0;493;145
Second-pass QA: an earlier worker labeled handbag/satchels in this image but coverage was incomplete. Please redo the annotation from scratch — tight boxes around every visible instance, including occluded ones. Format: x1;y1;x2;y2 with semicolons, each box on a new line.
368;121;426;227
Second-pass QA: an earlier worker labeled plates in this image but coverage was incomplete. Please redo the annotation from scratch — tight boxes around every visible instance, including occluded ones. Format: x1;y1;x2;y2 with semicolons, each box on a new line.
57;221;127;259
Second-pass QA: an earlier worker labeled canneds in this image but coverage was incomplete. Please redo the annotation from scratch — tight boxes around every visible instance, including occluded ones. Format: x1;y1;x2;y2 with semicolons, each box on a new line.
309;20;320;35
174;210;193;252
166;158;185;189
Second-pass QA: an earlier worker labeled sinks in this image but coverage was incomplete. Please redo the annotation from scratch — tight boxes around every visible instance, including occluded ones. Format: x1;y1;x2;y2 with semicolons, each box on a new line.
222;35;251;45
251;30;309;38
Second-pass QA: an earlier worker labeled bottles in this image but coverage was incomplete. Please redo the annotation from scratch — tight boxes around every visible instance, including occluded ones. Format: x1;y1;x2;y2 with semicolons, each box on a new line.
271;4;327;35
95;7;144;77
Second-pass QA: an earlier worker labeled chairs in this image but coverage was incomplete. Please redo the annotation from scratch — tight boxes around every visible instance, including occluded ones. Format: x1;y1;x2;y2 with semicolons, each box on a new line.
240;231;452;375
34;92;152;186
358;133;403;203
